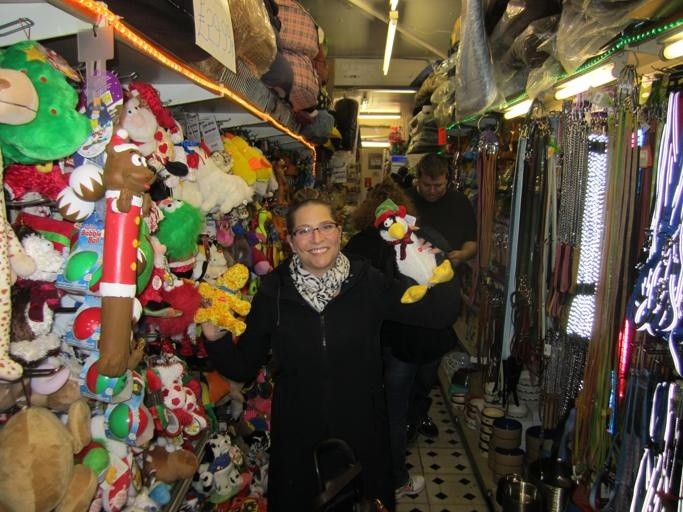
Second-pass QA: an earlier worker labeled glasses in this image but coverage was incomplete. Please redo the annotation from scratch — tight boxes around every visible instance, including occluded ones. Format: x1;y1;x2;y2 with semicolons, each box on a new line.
292;222;338;238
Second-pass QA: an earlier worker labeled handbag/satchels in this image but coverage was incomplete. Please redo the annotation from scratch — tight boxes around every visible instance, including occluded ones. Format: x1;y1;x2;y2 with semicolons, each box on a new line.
310;439;375;512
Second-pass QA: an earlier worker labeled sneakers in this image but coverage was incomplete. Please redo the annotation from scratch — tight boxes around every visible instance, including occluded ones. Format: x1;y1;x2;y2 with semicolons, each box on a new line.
394;476;425;500
406;416;437;442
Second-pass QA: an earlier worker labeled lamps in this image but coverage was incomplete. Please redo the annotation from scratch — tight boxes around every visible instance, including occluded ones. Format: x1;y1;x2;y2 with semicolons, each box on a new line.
658;31;683;62
381;10;400;78
500;92;545;120
553;62;625;102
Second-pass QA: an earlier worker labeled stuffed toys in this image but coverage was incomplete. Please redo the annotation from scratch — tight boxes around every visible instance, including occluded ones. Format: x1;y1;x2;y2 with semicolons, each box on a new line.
371;197;455;307
0;30;337;512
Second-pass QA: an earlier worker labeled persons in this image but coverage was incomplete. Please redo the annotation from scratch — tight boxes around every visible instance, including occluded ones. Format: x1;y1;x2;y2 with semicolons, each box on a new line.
332;179;457;500
199;183;463;512
391;152;481;442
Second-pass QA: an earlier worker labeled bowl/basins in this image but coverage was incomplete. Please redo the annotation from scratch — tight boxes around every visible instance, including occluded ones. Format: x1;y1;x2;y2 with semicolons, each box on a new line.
441;351;541;484
